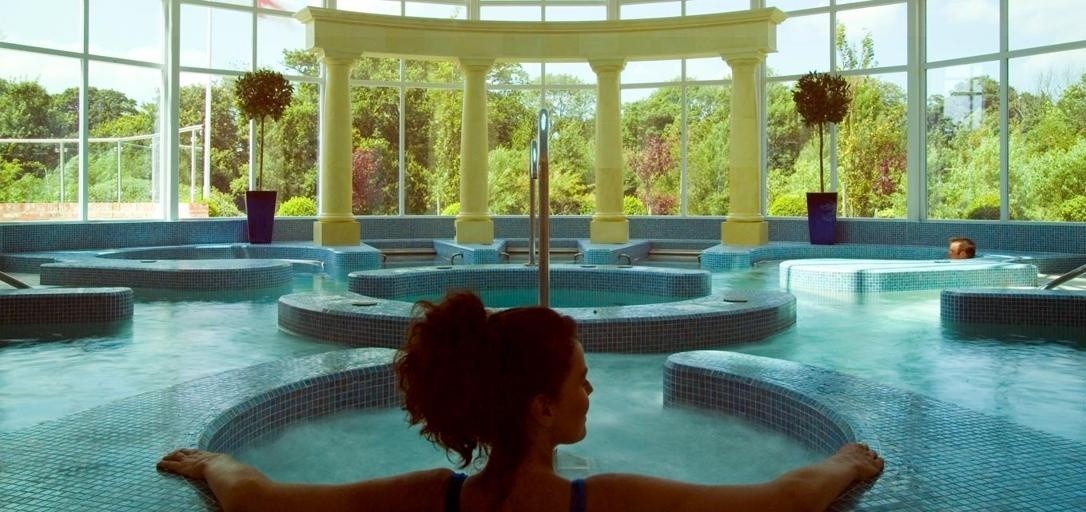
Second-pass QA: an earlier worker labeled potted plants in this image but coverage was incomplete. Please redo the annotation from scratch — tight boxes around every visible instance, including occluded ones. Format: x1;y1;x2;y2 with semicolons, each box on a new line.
789;69;853;245
234;67;295;244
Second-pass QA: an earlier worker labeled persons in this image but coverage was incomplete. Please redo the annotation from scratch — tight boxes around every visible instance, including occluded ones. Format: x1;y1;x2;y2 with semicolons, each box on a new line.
946;236;978;259
155;287;888;512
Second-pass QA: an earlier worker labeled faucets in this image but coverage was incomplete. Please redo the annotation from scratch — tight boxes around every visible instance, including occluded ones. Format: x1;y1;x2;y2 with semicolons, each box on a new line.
524;139;539;266
537;108;550;310
